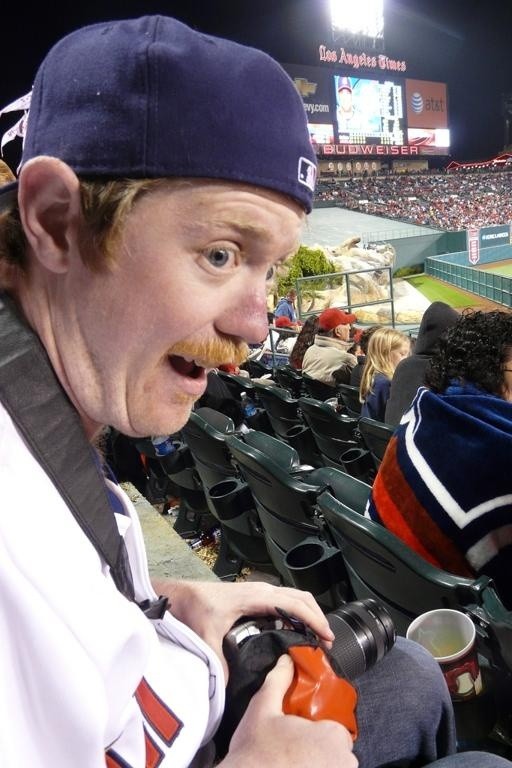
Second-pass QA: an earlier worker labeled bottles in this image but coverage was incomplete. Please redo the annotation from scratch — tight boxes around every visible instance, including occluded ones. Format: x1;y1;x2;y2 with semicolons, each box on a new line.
239;391;257;416
190;526;223;550
150;433;175;454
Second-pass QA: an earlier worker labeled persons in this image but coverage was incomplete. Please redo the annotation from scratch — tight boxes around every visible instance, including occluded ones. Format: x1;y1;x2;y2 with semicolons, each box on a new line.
1;13;510;768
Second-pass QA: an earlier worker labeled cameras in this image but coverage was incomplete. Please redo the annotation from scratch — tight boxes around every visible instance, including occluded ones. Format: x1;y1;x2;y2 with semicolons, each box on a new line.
222;597;397;731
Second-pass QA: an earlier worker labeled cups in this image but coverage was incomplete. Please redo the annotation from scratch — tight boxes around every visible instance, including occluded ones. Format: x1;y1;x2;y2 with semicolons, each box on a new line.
406;607;481;702
261;373;272;382
325;396;339;407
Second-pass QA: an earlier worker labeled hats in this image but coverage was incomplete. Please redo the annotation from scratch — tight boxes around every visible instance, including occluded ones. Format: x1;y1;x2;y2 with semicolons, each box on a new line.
317;307;357;332
276;316;293;328
16;13;319;214
